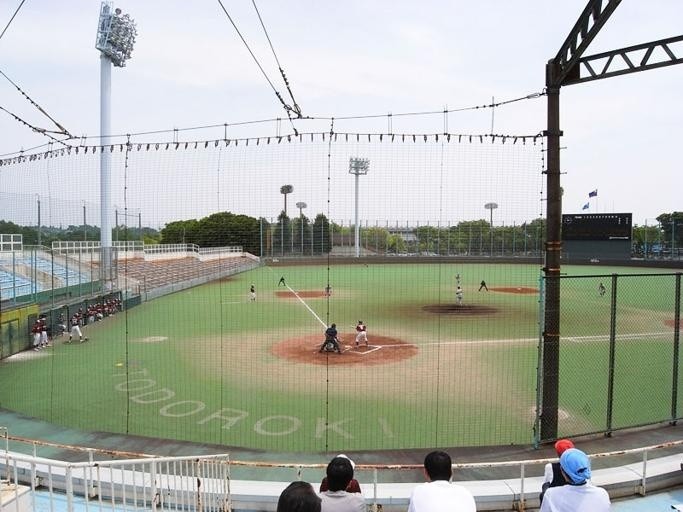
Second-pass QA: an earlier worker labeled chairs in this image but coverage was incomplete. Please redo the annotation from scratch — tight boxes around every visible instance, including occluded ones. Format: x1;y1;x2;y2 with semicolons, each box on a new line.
0;248;260;301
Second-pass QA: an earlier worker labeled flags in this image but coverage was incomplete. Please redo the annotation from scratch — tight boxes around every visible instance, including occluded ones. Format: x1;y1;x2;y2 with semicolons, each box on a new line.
588;189;596;198
580;202;588;211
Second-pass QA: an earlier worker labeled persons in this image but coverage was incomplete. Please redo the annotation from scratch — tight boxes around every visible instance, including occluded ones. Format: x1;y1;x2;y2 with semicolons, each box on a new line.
477;279;488;292
536;448;612;512
316;456;367;511
454;285;463;307
318;452;361;493
597;283;607;296
404;449;477;512
277;276;285;287
29;298;123;352
271;480;320;511
324;336;341;353
354;320;369;348
454;271;462;286
540;436;576;502
249;284;256;302
317;323;342;354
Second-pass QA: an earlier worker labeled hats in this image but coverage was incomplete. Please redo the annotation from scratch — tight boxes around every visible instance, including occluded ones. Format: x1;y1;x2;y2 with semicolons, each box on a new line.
555;440;591;485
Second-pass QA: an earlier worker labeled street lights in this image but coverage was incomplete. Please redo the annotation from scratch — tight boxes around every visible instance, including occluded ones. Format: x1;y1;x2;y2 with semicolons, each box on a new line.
484;203;498;256
295;201;307;218
92;1;138;296
280;184;293;213
348;155;369;257
